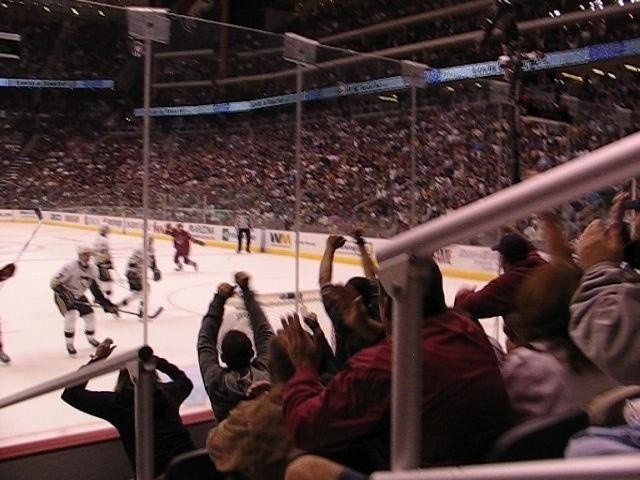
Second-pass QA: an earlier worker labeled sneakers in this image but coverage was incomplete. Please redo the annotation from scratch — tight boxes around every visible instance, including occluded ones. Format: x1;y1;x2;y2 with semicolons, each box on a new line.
237;249;252;254
88;337;100;347
175;263;199;271
1;352;11;363
66;343;77;354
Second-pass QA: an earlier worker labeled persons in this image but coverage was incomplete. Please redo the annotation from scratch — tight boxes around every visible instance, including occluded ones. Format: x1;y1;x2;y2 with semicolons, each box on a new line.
0;264;15;363
50;245;117;355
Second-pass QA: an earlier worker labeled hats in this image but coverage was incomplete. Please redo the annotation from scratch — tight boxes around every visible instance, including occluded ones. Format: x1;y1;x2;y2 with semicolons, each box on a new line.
76;244;91;255
491;234;532;254
99;223;112;234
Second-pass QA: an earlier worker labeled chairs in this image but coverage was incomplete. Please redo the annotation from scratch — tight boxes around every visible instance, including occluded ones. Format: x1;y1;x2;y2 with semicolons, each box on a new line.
585;379;639;432
484;405;590;461
161;444;216;480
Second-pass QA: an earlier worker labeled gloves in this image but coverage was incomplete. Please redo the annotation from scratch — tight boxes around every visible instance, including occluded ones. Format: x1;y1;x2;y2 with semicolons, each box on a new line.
0;262;15;282
53;285;76;310
152;269;161;282
101;299;119;315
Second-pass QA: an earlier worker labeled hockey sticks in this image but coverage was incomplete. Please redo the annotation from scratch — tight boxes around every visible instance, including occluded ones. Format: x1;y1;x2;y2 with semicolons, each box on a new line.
74;301;163;318
13;206;43;262
